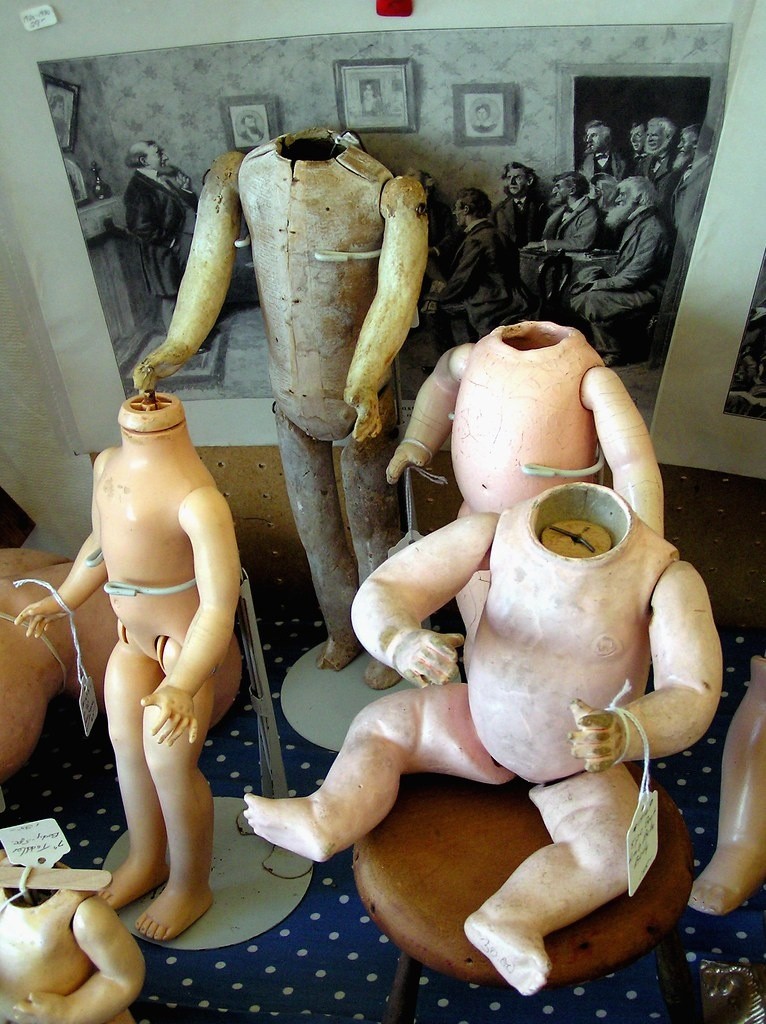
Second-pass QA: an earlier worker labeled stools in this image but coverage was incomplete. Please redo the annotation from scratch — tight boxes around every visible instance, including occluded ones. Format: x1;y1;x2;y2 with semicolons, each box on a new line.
351;769;698;1024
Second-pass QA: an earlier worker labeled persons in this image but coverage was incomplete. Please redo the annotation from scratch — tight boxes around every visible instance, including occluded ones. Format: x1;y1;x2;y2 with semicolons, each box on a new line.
0;388;244;1024
242;318;766;996
131;104;432;692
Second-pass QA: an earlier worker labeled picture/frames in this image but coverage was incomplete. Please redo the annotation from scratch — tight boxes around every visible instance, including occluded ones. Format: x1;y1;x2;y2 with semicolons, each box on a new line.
333;57;417;135
217;94;280;155
41;73;82;154
452;83;518;149
